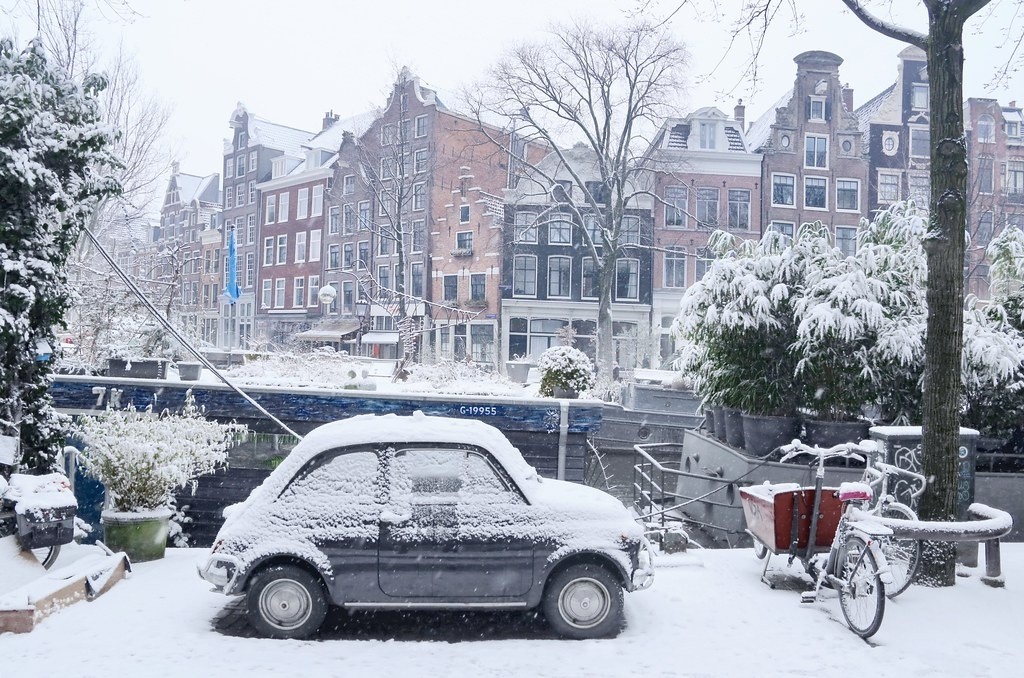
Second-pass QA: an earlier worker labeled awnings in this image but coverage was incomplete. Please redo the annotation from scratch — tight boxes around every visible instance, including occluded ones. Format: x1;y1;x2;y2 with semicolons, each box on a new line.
293;320;367;342
343;331;402;344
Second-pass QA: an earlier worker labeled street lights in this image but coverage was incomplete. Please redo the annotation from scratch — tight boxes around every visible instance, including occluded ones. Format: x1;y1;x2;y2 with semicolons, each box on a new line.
353;294;369;355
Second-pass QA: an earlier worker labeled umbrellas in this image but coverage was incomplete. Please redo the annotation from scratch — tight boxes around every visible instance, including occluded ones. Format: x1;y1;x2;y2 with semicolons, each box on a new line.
218;225;243;369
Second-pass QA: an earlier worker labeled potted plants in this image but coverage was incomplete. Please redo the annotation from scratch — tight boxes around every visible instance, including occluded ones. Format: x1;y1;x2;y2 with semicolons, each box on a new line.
538;345;596;399
177;316;202;381
668;199;1023;471
63;389;257;565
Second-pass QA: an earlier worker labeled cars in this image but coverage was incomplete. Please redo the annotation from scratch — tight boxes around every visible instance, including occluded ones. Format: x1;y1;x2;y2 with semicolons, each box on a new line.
198;417;656;640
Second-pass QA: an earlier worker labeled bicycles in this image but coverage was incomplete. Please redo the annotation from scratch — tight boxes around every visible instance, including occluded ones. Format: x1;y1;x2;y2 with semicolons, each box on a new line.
738;440;928;639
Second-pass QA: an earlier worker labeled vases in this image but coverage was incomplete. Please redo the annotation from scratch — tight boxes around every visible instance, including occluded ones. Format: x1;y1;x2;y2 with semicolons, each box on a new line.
107;357;170;379
506;362;531;383
